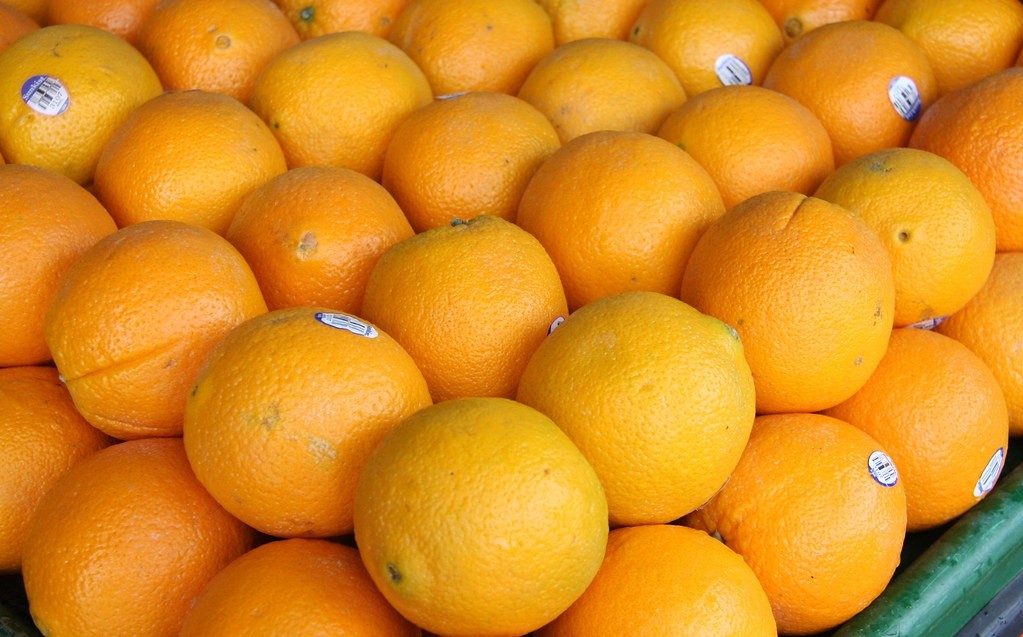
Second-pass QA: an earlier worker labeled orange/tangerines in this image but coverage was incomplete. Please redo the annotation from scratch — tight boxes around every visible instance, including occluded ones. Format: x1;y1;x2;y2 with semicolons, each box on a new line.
0;0;1023;637
